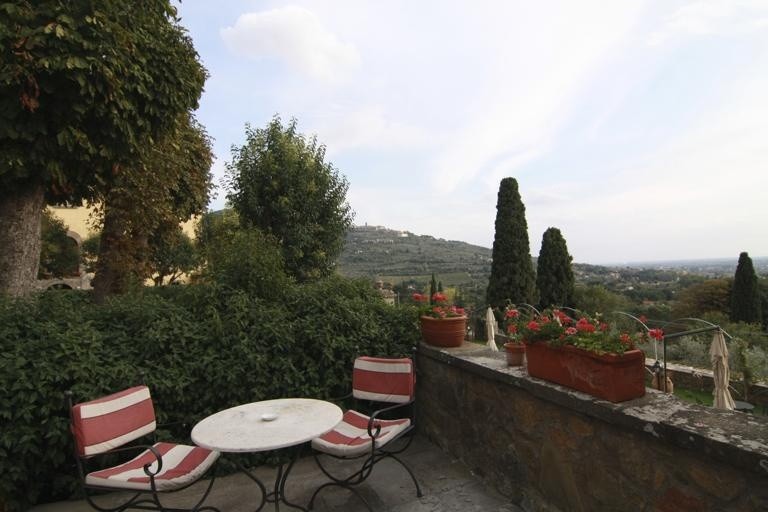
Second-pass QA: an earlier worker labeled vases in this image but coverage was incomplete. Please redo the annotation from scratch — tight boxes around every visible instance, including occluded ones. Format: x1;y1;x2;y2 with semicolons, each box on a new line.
524;338;647;404
418;315;467;347
503;341;523;365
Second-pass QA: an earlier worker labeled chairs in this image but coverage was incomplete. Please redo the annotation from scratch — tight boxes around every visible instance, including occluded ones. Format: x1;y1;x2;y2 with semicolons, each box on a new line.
68;383;223;512
307;352;423;512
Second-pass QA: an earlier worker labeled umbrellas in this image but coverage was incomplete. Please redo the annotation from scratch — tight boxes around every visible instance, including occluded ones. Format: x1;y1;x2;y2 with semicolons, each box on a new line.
484;303;500;353
708;324;736;409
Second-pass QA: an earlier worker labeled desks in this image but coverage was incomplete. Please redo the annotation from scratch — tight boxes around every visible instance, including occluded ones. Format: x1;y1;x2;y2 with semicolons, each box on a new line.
188;398;346;511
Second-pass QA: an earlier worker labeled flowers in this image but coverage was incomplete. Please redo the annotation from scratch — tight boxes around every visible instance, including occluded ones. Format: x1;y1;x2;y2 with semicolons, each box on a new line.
410;293;465;320
499;299;663;358
